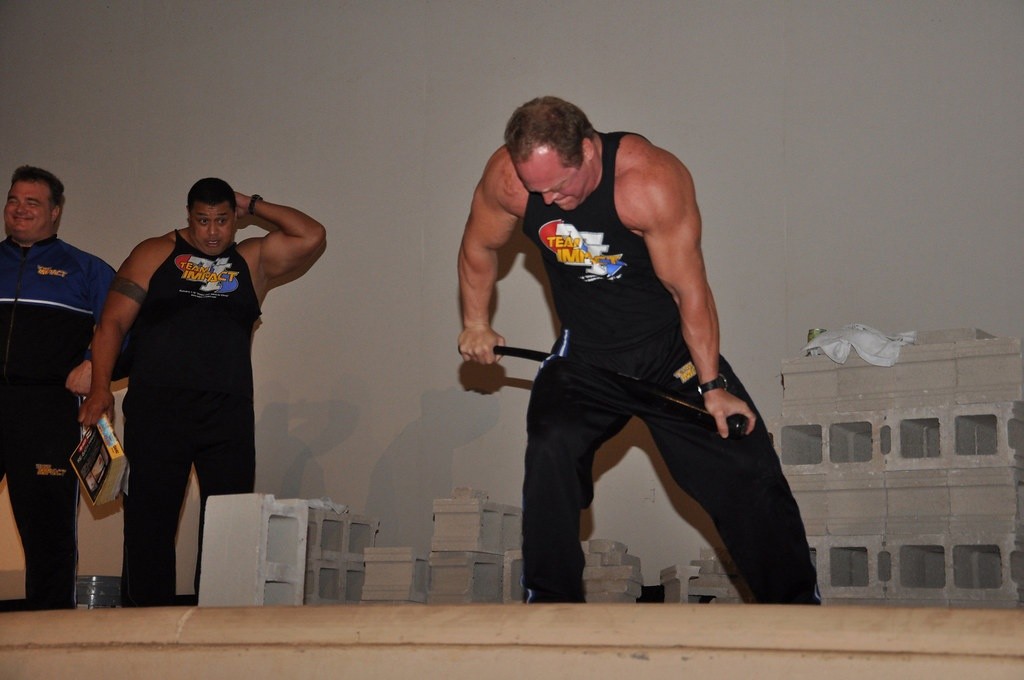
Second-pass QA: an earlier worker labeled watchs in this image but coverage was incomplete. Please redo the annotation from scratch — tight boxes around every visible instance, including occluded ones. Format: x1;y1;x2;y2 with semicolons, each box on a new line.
697;373;727;396
248;194;263;216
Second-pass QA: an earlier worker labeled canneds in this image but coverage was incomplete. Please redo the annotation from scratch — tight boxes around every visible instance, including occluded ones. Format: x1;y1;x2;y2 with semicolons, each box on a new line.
808;328;827;343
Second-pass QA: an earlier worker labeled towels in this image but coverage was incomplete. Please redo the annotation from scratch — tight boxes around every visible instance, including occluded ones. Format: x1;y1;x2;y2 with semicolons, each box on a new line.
308;495;351;515
799;323;918;367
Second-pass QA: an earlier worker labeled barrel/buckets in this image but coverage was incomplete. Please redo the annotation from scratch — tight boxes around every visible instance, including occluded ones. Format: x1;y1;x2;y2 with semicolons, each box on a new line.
75;573;121;608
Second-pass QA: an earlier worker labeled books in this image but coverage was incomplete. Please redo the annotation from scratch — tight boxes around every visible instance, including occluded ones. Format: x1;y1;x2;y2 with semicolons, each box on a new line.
69;412;130;506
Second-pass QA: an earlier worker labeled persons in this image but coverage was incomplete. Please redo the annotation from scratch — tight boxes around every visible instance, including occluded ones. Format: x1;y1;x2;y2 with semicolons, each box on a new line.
455;94;825;604
0;165;328;611
